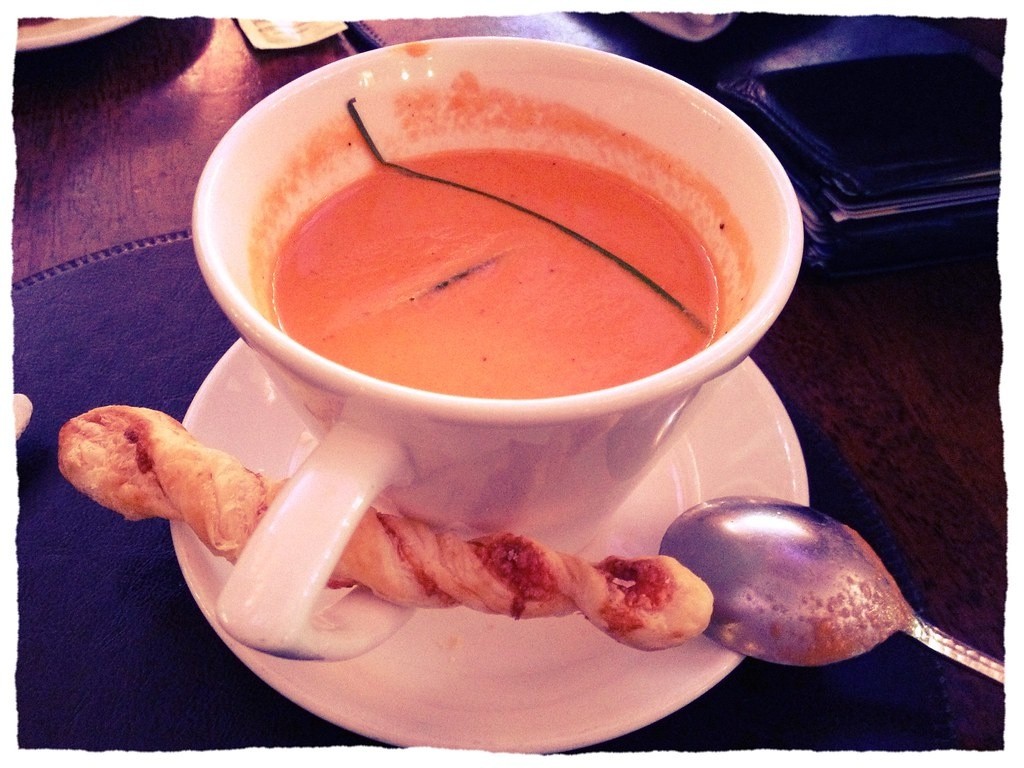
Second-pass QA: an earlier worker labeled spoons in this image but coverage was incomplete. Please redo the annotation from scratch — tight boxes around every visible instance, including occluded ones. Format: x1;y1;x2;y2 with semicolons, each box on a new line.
655;496;1004;697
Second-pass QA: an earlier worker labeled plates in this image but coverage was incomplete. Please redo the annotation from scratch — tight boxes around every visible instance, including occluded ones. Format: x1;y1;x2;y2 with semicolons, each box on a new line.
16;17;140;50
168;324;814;755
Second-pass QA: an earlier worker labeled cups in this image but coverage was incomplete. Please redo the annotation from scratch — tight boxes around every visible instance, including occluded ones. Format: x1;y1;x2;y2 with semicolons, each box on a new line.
191;36;804;662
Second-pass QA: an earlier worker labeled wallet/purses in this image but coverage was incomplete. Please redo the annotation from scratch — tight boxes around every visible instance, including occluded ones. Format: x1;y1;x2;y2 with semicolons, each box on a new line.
716;52;1001;280
